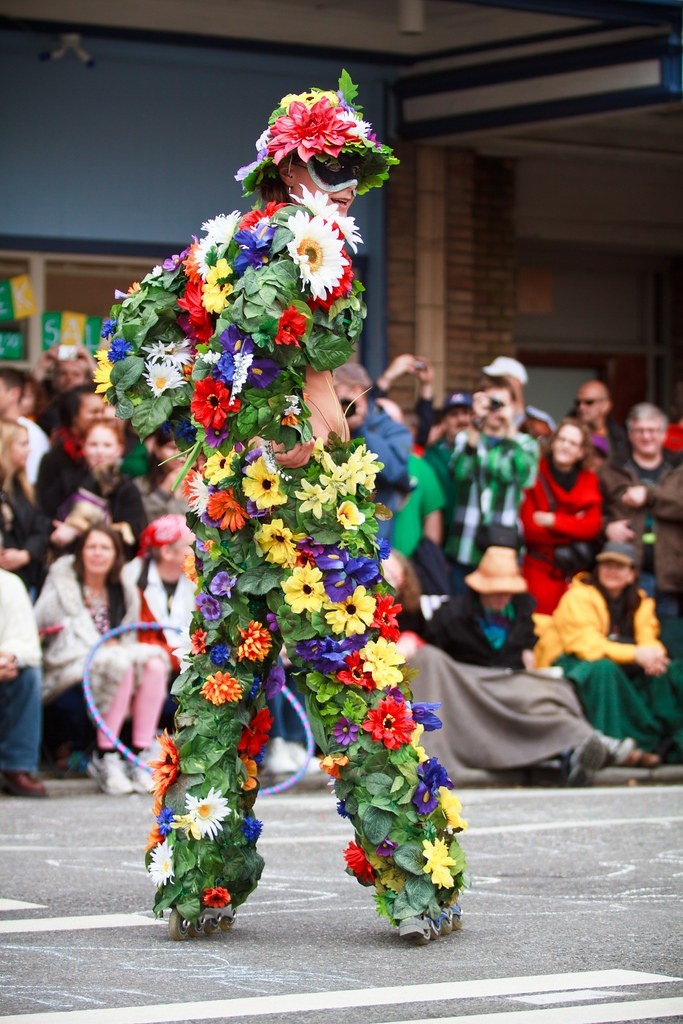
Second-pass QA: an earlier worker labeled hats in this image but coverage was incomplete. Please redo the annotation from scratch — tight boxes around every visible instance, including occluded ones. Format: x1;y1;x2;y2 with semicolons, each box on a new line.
482;354;530;388
596;543;640;570
441;391;472;416
465;545;526;593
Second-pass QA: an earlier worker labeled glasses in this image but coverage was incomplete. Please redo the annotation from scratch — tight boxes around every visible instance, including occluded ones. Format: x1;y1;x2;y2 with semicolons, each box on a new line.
572;397;608;407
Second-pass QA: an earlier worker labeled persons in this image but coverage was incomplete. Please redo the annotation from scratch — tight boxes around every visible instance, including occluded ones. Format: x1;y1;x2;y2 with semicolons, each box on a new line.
0;340;683;797
91;68;465;942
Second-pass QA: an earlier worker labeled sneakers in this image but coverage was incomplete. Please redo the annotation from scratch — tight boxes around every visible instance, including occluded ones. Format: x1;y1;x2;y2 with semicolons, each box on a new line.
86;748;137;796
125;760;159;793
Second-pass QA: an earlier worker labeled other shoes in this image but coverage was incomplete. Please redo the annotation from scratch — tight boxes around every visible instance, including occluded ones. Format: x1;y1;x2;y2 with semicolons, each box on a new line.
560;734;607;788
600;731;636;771
0;767;46;797
265;735;299;775
628;749;661;770
289;741;322;771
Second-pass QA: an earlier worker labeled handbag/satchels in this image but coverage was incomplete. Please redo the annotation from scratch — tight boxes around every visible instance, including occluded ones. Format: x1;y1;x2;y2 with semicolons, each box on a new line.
553;543;594;573
474;524;520;556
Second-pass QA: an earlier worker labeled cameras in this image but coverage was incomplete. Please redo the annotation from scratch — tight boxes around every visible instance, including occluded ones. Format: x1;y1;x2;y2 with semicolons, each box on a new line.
485;396;503;410
414;360;426;370
